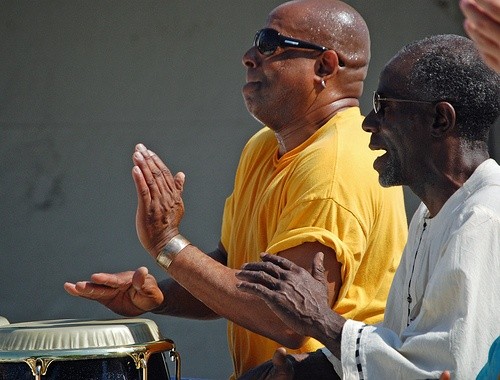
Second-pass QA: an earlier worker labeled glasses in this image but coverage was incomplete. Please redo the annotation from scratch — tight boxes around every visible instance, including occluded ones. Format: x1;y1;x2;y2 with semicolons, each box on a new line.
372;90;438;115
252;28;345;68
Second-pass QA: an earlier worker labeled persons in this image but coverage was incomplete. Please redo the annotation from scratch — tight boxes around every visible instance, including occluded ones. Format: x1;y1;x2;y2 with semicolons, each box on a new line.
457;0;500;76
229;34;500;380
63;0;410;380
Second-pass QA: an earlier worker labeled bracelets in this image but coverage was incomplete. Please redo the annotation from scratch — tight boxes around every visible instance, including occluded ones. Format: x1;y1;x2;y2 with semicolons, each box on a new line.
154;234;193;271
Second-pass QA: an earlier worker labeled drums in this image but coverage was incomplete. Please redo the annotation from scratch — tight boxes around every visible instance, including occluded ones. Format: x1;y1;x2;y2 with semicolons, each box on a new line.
0;317;181;379
